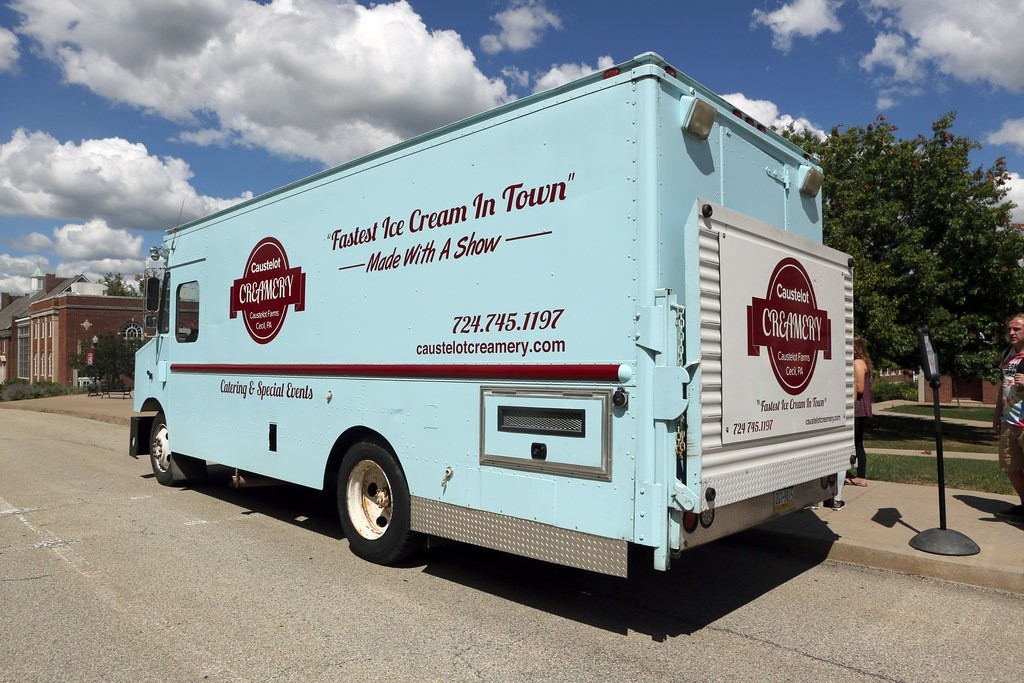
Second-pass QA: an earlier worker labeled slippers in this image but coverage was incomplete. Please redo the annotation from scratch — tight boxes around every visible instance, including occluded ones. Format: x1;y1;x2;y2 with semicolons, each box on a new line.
844;477;867;487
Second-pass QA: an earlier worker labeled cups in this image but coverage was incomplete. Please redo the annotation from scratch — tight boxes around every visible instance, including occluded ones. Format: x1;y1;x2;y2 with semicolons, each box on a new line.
1016;373;1023;387
1002;369;1016;386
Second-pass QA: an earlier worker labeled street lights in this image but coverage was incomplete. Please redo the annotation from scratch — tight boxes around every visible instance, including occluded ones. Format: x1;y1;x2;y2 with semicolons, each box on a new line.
93;335;99;392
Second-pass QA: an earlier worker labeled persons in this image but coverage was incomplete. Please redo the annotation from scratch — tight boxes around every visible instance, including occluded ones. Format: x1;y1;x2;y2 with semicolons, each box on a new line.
995;314;1024;527
842;334;871;486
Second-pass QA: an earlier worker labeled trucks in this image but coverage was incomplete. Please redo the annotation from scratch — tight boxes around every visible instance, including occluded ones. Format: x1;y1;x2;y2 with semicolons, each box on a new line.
127;48;859;581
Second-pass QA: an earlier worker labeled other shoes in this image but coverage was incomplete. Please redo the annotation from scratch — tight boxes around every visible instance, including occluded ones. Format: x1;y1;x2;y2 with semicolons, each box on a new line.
997;505;1024;519
832;500;846;511
1006;515;1024;526
